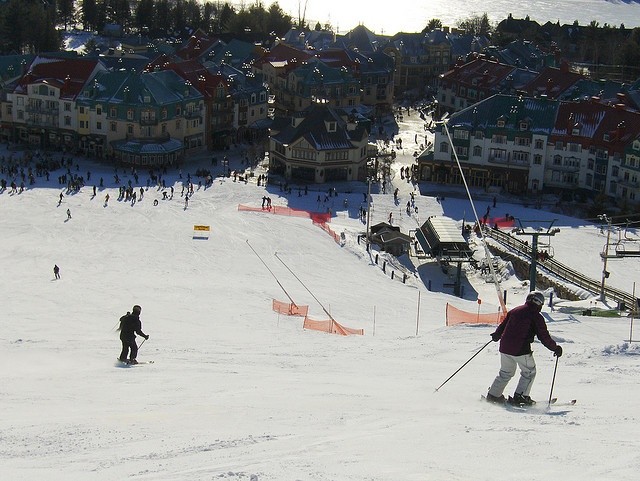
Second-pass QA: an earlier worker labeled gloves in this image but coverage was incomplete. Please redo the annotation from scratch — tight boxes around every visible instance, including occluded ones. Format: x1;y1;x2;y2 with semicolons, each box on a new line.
491;333;501;342
554;346;562;357
145;335;149;340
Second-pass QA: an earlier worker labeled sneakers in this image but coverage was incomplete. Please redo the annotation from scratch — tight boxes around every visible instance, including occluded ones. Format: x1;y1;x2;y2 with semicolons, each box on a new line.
120;358;129;363
130;358;138;364
514;392;536;406
487;393;508;405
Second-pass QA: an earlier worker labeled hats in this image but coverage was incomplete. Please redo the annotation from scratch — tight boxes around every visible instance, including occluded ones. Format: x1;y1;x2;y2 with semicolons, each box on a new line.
133;305;141;313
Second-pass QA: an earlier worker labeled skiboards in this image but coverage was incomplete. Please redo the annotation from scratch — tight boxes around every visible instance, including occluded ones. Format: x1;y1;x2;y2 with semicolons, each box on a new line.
114;358;155;370
480;394;578;414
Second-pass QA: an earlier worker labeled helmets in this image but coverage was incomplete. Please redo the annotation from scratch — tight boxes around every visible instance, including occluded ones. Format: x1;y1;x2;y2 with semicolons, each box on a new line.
527;291;544;306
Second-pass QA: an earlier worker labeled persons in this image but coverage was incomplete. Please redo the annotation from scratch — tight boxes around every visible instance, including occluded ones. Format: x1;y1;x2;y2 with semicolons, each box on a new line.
116;312;130;331
378;95;435;178
486;291;561;407
223;145;274;211
53;265;60;280
278;180;418;224
119;306;149;364
482;195;515;230
0;149;219;221
